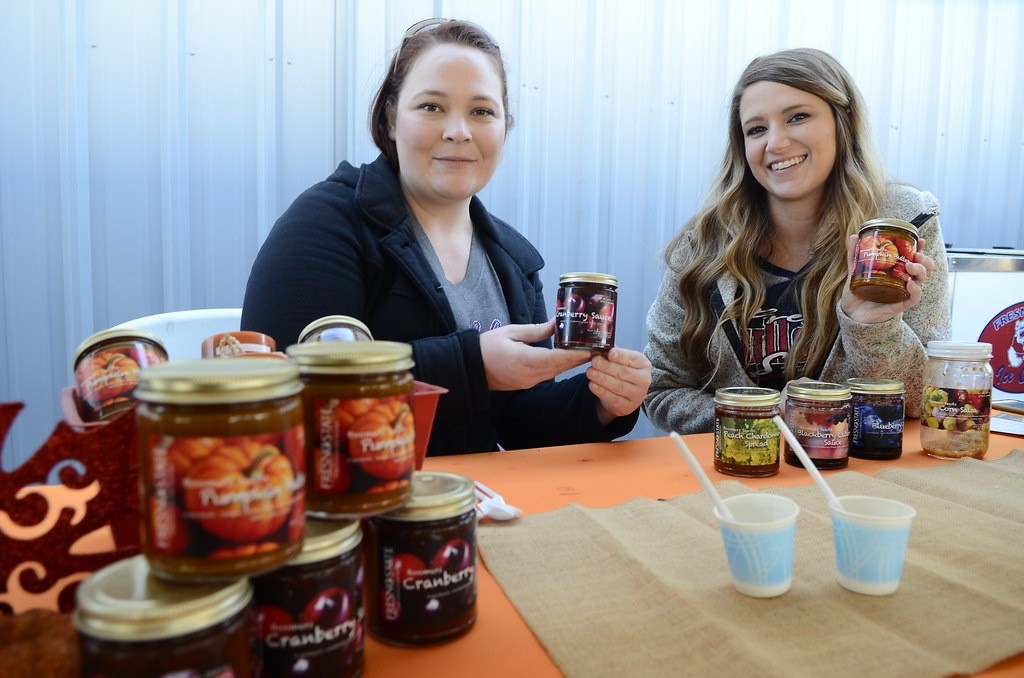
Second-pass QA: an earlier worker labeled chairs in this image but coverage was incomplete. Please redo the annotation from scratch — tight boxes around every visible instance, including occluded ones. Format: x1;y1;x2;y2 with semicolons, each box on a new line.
76;306;242;362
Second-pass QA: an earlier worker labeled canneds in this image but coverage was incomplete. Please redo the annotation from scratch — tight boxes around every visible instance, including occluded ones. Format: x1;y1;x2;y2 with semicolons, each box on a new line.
848;218;921;303
919;341;994;461
71;554;264;678
72;326;172;428
712;387;781;477
553;271;618;351
843;377;906;460
784;381;853;470
134;314;477;678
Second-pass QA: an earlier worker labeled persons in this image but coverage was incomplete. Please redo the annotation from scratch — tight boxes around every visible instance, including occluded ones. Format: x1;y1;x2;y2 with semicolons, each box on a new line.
641;47;952;434
239;18;652;457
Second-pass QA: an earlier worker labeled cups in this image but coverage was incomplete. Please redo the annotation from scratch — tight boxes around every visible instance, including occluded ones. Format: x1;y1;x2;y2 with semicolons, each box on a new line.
713;493;800;599
829;495;917;596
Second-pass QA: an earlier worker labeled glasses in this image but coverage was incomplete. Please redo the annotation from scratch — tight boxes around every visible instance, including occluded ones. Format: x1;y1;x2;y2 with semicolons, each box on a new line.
393;18;500;75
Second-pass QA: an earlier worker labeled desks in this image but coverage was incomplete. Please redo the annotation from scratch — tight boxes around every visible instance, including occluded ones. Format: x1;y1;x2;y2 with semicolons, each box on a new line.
362;409;1024;678
